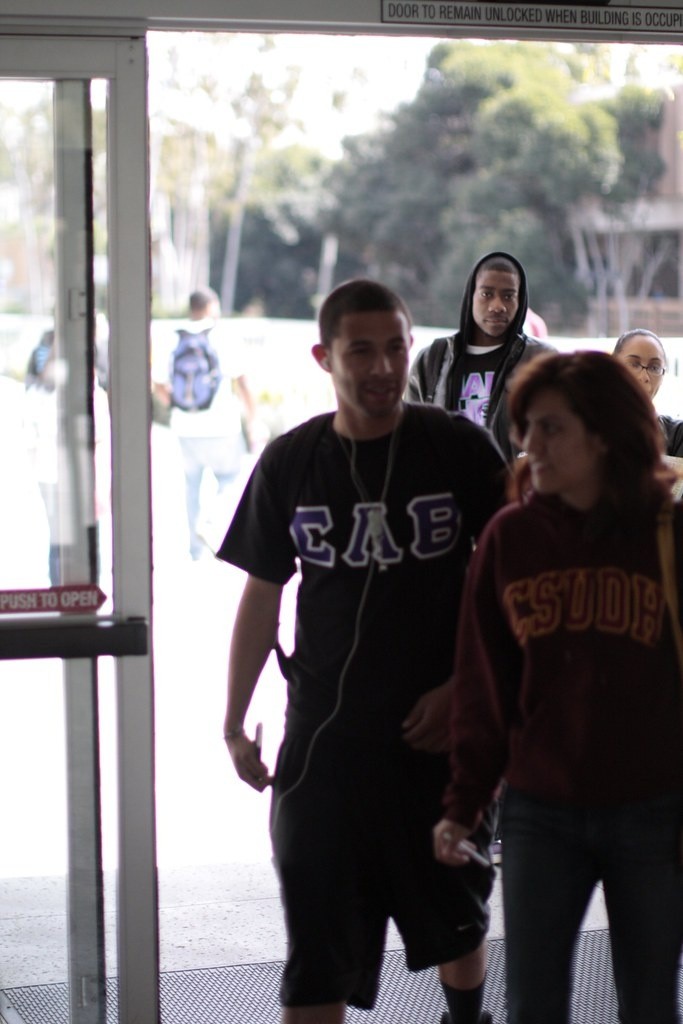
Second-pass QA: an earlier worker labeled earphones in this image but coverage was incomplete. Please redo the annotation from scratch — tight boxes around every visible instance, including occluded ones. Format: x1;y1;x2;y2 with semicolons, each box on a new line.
321;356;331;372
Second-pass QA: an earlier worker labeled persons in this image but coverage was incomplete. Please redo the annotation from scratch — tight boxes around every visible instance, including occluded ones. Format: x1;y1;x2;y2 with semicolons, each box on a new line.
19;252;683;599
213;277;513;1024
447;350;683;1024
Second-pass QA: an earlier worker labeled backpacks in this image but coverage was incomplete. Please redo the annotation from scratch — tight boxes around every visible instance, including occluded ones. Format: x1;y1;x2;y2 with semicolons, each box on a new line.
169;327;221;413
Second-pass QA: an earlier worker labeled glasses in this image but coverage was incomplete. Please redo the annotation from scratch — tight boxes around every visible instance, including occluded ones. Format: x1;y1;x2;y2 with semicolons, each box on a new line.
625;362;665;377
